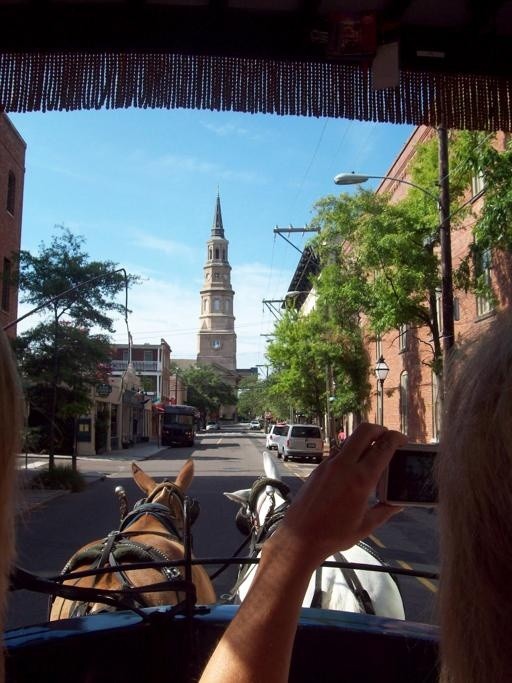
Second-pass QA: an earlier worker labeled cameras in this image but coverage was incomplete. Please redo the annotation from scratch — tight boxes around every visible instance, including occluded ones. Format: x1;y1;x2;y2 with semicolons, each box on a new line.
376;443;444;509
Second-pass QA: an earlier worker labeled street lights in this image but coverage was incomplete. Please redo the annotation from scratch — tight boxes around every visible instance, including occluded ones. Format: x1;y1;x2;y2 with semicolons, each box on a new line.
373;354;389;427
333;172;455;400
287;291;318;297
266;339;277;342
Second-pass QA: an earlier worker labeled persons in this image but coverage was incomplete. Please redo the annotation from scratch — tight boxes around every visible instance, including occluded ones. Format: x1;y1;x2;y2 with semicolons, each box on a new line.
338;428;345;445
192;304;511;681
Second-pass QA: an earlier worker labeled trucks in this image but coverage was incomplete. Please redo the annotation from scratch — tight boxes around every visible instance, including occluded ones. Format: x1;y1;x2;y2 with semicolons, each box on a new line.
160;405;200;447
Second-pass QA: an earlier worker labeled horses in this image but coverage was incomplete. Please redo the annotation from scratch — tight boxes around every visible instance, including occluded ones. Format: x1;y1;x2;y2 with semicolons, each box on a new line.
221;447;407;622
49;454;217;624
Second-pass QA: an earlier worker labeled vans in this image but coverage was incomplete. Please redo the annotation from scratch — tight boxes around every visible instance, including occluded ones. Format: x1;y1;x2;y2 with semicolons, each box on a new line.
264;425;323;463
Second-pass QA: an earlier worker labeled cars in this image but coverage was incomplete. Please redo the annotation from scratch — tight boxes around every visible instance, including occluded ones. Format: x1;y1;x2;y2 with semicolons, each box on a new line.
249;420;260;430
206;421;218;431
